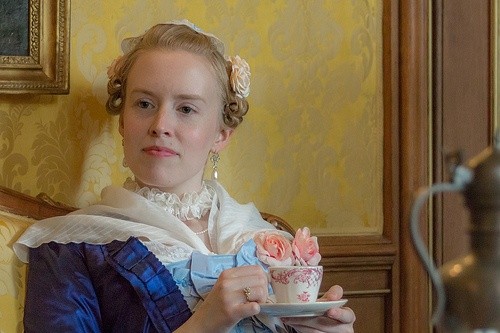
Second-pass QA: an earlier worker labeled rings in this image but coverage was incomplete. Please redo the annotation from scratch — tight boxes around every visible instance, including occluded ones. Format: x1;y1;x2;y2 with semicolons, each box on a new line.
243;288;250;301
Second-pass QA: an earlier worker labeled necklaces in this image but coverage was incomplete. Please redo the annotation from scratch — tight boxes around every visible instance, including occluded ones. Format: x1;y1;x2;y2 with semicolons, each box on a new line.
193;227;208;235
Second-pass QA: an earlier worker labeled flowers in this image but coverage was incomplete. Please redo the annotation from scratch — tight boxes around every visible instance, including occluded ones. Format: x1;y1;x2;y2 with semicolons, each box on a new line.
254;231;295;267
292;226;322;266
230;56;251;99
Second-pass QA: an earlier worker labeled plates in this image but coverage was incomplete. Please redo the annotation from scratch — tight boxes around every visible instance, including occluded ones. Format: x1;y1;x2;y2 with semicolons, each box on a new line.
260;299;348;317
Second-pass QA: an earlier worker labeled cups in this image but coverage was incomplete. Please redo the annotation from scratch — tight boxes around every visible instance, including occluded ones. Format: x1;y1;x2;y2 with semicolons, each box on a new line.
266;266;323;302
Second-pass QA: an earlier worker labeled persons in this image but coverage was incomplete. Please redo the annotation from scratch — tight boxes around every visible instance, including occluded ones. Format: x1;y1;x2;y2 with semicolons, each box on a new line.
12;19;356;333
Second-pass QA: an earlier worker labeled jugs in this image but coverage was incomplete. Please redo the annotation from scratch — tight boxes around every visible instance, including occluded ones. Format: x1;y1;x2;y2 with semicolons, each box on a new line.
404;128;500;333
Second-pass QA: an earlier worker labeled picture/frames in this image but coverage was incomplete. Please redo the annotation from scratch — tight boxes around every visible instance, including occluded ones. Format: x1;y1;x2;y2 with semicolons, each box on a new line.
0;0;71;95
